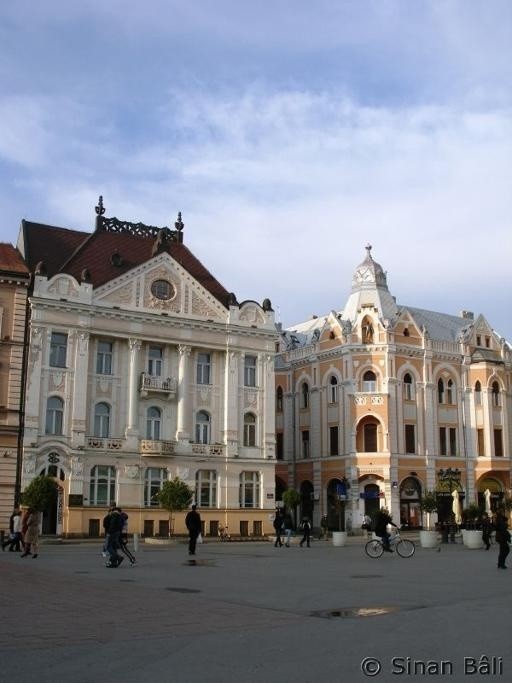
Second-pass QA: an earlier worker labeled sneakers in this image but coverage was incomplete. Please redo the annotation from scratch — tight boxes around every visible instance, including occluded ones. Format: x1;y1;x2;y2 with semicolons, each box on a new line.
498;563;507;569
486;544;490;550
189;551;196;556
275;543;290;547
299;543;311;547
102;551;135;568
1;544;37;559
386;548;394;553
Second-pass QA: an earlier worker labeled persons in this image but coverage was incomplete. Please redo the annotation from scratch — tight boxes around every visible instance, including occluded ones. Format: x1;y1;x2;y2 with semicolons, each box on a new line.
318;513;329;540
1;500;42;558
185;504;202;555
101;503;136;567
481;511;491;550
363;512;372;530
375;508;400;552
273;510;284;547
300;517;311;547
495;515;511;569
283;512;294;547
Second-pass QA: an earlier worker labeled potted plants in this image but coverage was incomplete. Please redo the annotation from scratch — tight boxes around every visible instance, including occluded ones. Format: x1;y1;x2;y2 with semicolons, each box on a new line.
418;492;440;550
460;505;486;550
328;489;349;547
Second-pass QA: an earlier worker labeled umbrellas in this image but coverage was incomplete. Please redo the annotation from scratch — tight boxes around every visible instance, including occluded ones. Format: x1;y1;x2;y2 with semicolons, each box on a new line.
452;489;463;526
484;489;495;519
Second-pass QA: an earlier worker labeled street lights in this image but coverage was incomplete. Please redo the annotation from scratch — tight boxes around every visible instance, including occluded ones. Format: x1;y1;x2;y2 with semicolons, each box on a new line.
437;467;462;542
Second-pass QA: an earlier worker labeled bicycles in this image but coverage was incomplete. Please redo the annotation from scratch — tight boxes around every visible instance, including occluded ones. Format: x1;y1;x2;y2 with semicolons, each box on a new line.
364;523;416;558
217;522;233;544
